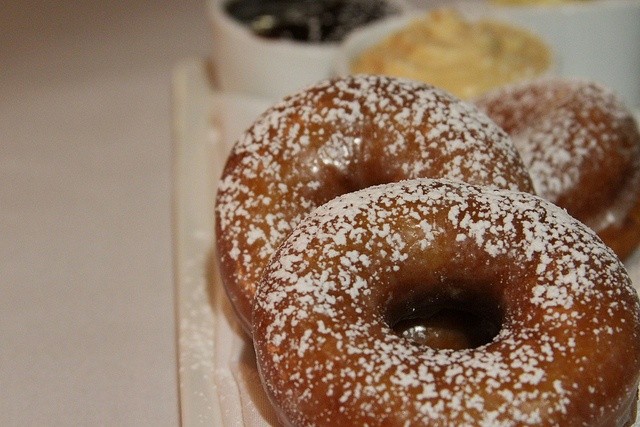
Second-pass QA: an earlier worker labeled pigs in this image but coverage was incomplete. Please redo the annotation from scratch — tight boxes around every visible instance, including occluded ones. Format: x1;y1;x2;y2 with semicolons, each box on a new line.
471;79;640;260
215;73;537;339
250;177;635;426
